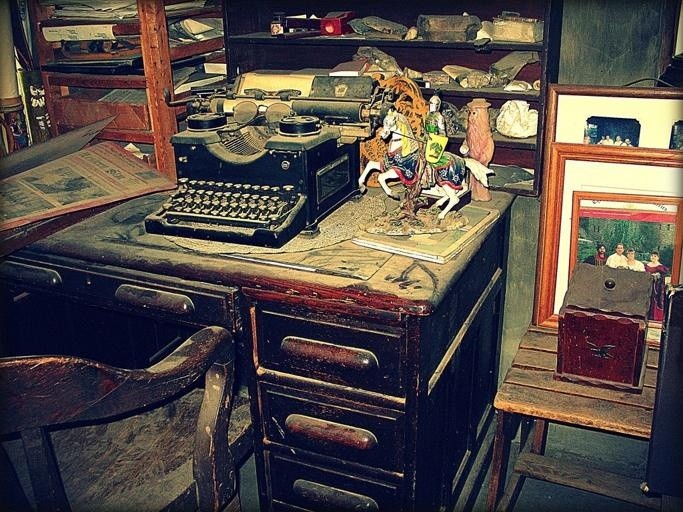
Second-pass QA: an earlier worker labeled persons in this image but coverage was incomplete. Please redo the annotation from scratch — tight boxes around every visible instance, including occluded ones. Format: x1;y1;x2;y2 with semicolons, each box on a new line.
644;251;672;321
582;243;607;265
596;133;636;147
601;243;630;272
623;246;645;271
420;95;448;176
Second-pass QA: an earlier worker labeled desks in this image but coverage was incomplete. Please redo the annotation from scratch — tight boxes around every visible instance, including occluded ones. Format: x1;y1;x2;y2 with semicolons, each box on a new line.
1;189;517;511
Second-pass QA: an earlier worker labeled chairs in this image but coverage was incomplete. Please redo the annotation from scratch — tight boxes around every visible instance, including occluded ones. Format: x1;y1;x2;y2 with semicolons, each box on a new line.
3;326;253;512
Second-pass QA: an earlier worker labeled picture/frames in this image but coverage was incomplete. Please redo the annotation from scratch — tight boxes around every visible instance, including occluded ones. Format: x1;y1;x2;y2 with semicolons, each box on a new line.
542;82;683;219
538;141;683;351
569;190;683;329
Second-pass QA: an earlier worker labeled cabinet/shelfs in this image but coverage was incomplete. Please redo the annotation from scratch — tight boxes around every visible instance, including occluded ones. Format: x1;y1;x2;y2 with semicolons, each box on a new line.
222;1;564;194
33;1;228;183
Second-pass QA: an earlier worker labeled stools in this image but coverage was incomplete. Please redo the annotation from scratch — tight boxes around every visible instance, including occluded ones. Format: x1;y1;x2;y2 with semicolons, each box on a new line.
485;324;659;512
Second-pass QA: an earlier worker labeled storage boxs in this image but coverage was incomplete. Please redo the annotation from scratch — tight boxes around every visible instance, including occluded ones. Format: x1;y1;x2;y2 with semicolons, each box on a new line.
554;263;654;393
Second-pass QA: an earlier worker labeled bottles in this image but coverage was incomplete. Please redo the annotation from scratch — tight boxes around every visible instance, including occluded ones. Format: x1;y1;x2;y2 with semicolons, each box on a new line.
270;12;286;36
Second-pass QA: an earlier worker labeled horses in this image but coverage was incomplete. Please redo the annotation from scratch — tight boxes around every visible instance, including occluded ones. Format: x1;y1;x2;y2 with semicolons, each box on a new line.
358;109;495;225
